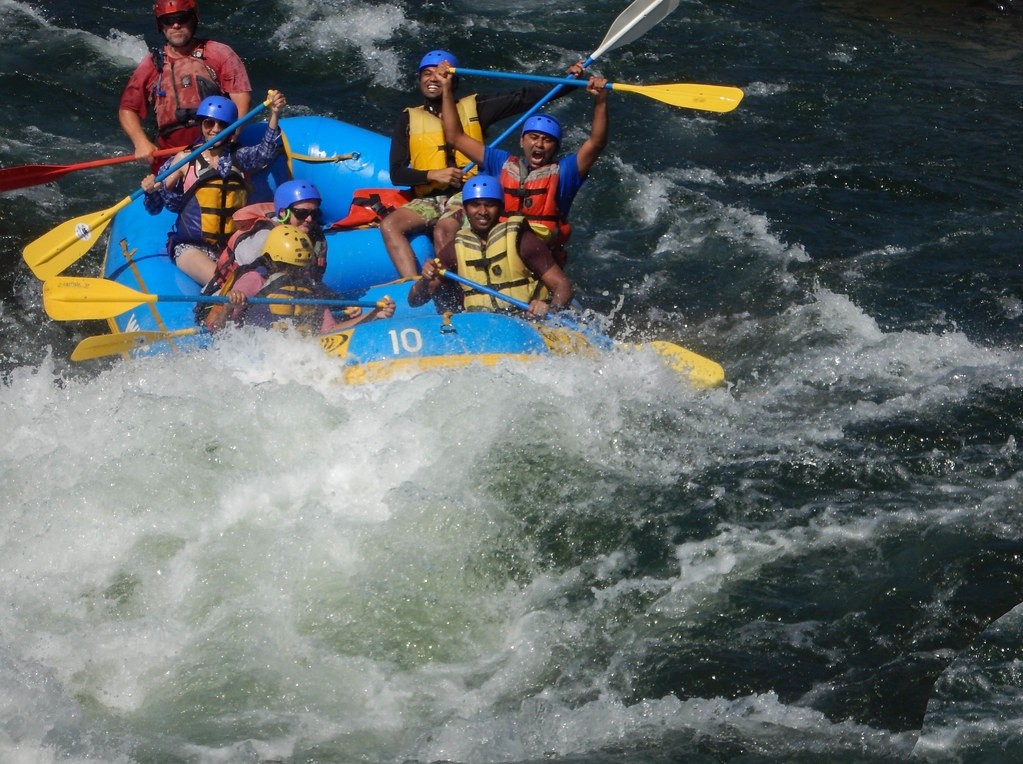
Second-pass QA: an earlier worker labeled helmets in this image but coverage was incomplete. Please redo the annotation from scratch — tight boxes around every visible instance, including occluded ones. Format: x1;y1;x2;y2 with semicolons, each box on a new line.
462;174;504;205
196;95;238;124
417;50;458;71
274;179;321;219
151;0;202;34
521;113;563;141
261;224;314;267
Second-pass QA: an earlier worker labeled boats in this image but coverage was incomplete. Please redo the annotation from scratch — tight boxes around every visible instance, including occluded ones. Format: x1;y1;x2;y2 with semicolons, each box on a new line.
98;116;605;391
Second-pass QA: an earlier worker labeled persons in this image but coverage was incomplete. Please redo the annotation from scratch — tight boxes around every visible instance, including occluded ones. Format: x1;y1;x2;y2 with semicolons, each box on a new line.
142;90;286;287
434;60;609;269
407;176;574;323
194;178;395;342
117;0;252;174
381;51;585;277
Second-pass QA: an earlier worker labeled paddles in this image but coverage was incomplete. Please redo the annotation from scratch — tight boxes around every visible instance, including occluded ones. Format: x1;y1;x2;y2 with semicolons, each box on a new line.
462;0;683;173
23;89;276;282
432;257;726;390
1;145;186;194
447;65;745;113
41;276;391;323
69;306;361;362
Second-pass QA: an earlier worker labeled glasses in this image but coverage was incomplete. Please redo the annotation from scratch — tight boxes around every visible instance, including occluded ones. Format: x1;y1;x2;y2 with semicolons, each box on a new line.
289;207;323;220
202;118;229;129
161;13;193;25
